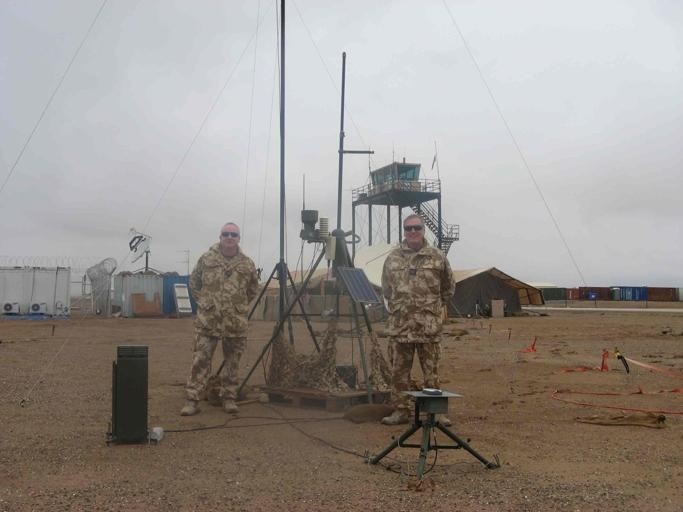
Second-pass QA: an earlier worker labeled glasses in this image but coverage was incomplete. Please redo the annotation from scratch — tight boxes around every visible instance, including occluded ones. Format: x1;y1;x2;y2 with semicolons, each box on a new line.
402;224;423;231
219;232;240;238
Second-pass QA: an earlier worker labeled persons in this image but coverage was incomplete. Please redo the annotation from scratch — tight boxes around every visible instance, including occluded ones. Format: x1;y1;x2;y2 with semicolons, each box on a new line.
381;214;454;427
177;221;259;417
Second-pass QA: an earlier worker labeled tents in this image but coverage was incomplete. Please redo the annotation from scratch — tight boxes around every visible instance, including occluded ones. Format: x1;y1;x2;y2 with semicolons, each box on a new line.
446;266;550;316
253;265;382;324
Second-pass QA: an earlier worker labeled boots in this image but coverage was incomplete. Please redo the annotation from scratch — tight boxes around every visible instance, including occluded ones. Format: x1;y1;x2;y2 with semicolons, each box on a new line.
380;408;409;426
222;397;239;413
179;400;201;417
433;412;451;427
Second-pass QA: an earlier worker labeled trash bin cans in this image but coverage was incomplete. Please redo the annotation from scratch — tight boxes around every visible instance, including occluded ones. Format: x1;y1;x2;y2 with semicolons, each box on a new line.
112;305;120;313
608;287;620;301
